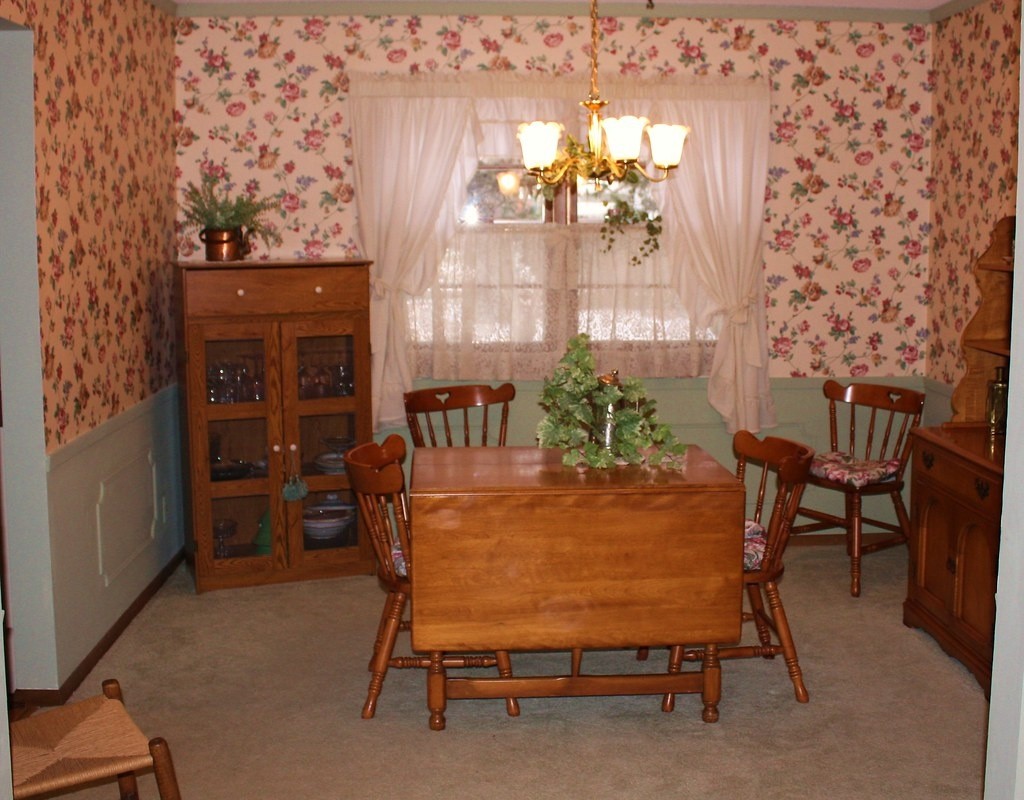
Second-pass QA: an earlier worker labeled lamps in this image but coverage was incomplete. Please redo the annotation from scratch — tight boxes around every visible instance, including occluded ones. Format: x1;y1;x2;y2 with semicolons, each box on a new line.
495;1;689;198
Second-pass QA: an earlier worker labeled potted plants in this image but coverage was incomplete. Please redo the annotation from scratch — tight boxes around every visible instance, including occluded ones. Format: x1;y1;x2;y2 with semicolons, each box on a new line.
176;177;276;262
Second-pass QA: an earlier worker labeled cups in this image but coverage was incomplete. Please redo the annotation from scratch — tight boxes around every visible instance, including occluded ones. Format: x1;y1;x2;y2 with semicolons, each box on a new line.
206;359;354;404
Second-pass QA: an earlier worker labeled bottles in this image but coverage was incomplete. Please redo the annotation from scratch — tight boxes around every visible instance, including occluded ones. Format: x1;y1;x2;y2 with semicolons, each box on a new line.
983;366;1008;435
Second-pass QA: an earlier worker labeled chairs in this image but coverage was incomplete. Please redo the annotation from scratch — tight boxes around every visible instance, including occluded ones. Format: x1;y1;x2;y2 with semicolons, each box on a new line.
637;430;815;723
404;382;516;447
790;380;926;597
344;434;520;718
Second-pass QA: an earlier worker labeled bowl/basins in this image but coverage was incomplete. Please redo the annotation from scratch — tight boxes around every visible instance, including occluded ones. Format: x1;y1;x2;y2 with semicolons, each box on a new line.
301;508;356;540
315;453;352;475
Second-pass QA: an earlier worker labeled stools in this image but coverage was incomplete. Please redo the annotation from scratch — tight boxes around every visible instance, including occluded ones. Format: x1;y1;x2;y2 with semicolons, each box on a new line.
10;679;181;800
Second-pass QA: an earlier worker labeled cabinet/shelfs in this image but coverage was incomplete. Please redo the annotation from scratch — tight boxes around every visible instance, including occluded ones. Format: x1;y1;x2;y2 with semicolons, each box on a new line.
903;215;1017;702
173;258;376;596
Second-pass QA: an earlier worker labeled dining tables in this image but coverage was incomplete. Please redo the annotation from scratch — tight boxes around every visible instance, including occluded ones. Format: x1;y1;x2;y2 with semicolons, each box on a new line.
410;444;746;731
